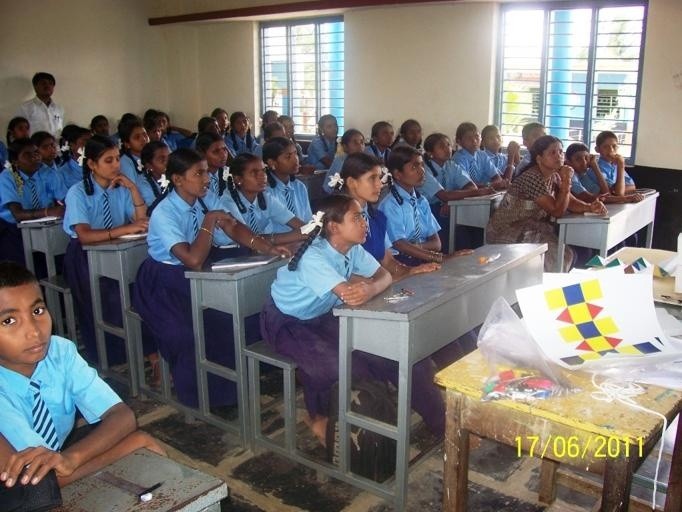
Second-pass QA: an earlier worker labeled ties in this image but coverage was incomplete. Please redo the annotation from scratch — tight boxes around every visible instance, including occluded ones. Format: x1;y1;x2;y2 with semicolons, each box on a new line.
103;193;113;229
210;177;219;195
27;380;60;453
248;205;257;234
362;214;369;234
344;256;350;280
191;209;198;242
469;160;477;183
284;187;296;214
29;180;40;210
441;169;447;190
410;197;420;238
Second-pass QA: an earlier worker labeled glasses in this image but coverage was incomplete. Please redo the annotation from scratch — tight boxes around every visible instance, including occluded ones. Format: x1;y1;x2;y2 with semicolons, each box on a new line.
250;167;267;177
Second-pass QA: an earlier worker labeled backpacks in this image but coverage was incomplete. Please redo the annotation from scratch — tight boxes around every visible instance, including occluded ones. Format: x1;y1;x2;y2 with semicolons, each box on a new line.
327;379;398;481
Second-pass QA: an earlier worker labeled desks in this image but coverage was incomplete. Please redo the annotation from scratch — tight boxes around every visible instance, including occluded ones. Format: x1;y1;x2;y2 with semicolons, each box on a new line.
15;155;681;511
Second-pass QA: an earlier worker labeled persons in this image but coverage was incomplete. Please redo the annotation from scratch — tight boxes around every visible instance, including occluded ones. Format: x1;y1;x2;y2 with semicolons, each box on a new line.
6;117;31;144
134;147;237;404
17;73;65;144
261;194;393;449
29;108;422;206
1;261;166;485
2;138;69;260
377;146;477;370
422;123;639;271
327;152;445;437
221;153;310;370
63;133;161;388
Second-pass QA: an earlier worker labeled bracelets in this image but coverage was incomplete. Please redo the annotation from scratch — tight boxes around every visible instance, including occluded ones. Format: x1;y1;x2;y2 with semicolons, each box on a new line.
32;209;36;218
251;236;263;248
133;200;146;207
199;225;214;235
45;209;47;217
107;230;111;240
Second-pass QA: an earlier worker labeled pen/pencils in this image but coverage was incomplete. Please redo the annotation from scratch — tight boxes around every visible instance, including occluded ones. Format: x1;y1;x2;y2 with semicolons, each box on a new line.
57;200;64;206
137;481;165;497
401;287;414;296
